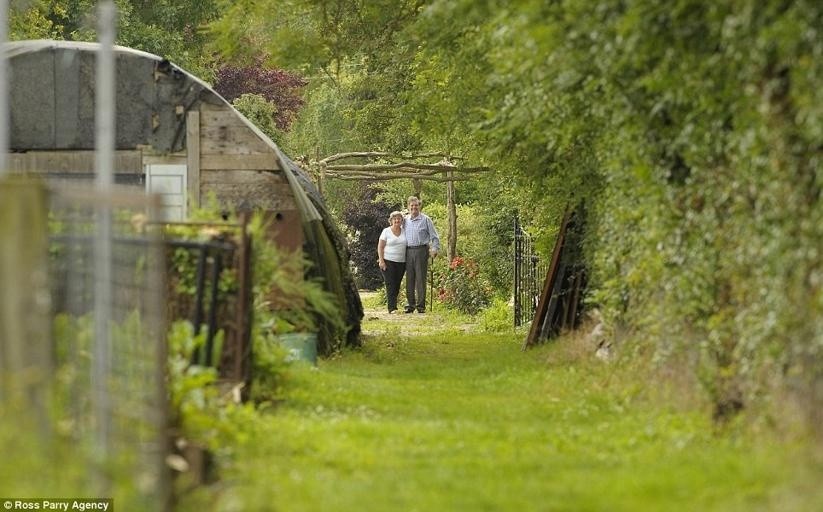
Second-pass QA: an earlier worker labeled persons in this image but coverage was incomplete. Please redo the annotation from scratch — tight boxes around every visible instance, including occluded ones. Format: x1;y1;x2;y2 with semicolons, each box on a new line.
401;196;439;313
377;211;407;314
